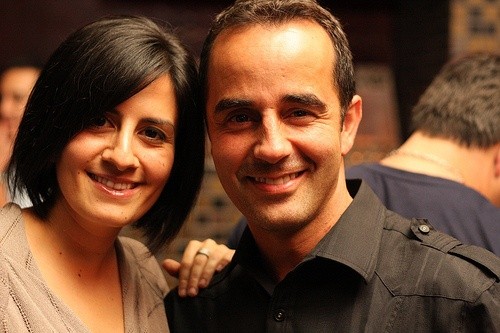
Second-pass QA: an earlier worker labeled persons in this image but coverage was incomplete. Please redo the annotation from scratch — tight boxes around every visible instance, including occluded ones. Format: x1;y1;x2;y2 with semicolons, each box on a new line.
0;67;43;209
224;49;500;256
161;0;499;333
0;18;236;333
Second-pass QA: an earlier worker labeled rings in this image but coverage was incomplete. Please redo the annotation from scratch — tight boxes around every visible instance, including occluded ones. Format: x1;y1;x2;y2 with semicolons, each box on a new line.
197;249;209;257
